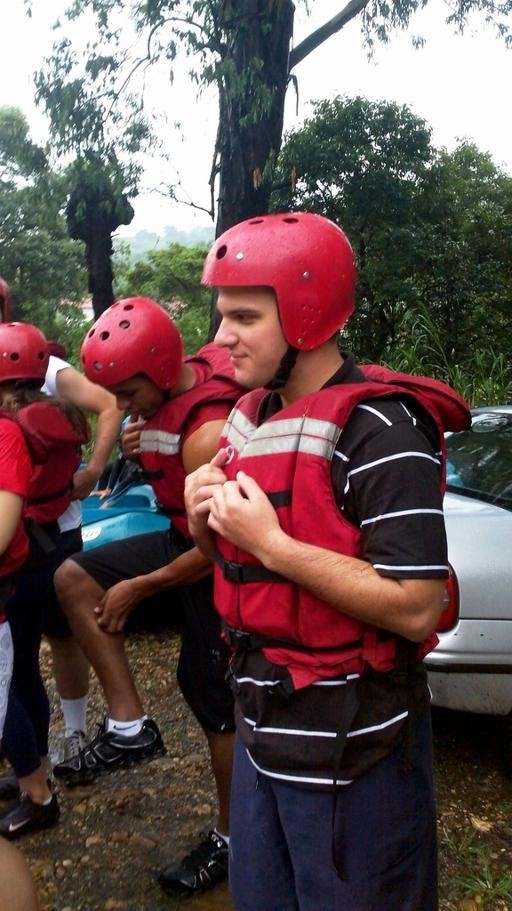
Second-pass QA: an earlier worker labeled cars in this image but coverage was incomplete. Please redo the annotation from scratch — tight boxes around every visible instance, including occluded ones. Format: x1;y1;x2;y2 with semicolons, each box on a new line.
422;405;512;719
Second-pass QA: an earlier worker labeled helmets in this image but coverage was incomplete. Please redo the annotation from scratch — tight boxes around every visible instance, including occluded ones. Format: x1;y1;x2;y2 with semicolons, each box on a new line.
80;296;184;392
199;212;357;353
0;277;69;389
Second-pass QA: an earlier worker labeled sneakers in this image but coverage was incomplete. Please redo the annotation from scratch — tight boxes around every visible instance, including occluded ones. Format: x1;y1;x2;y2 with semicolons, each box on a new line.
159;829;228;901
0;773;61;841
52;711;168;790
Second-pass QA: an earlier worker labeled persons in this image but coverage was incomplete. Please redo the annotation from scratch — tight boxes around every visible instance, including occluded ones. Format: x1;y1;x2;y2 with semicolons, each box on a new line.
2;281;127;911
178;203;477;909
49;295;254;903
